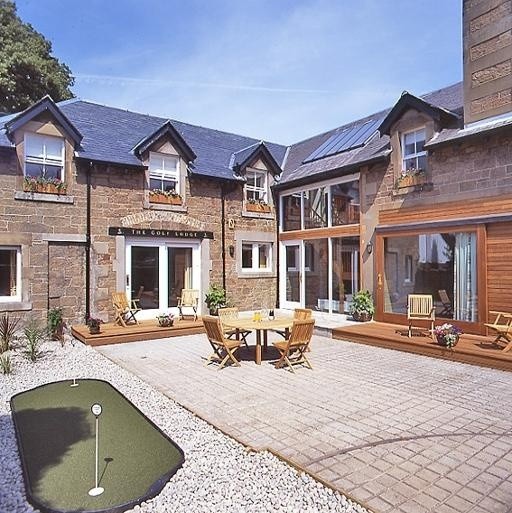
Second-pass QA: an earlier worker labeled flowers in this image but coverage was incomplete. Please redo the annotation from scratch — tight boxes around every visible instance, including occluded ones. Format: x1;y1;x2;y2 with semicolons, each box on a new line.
86;316;105;330
399;167;425;179
434;321;465;350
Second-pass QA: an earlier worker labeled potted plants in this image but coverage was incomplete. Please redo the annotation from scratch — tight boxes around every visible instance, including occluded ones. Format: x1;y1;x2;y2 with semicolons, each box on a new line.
204;282;227;317
347;286;377;321
148;188;183;205
246;198;272;213
22;174;68;195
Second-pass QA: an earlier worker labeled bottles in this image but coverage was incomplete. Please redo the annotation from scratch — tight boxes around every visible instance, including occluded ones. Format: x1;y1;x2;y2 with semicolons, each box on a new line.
254;303;275;322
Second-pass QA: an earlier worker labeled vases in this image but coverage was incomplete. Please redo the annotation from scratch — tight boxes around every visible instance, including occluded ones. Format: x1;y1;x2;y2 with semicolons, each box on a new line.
88;326;101;334
435;331;460;348
399;177;424;190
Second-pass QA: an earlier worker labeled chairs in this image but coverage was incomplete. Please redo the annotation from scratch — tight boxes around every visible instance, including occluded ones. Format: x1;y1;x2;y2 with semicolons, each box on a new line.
483;309;512;352
437;288;455;318
132;286;145;307
111;291;143;328
199;305;316;375
175;287;200;321
406;293;436;339
